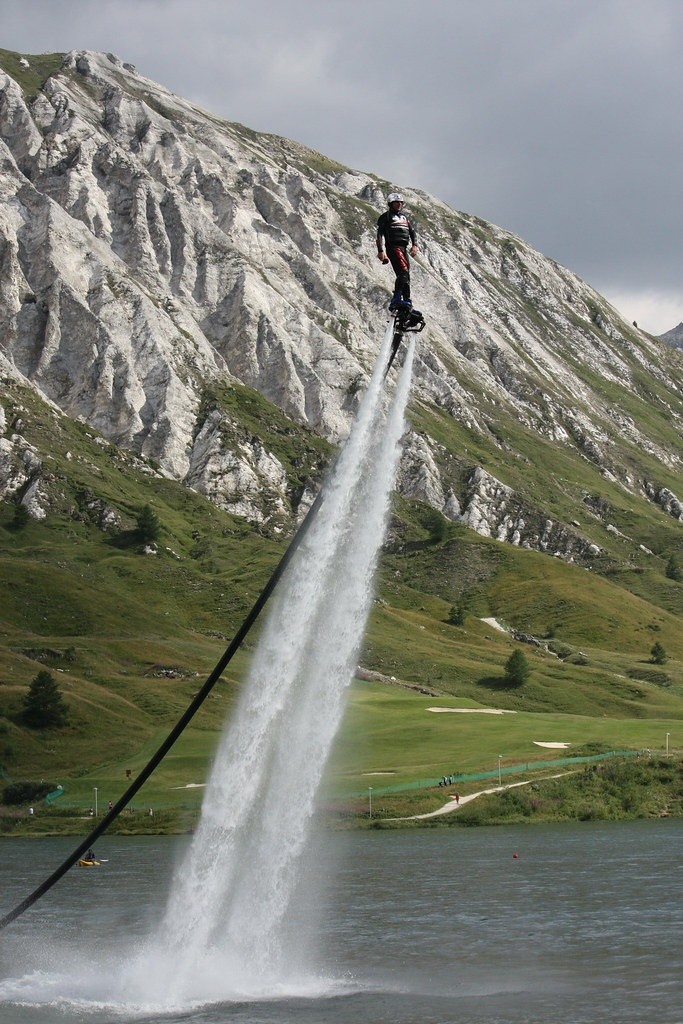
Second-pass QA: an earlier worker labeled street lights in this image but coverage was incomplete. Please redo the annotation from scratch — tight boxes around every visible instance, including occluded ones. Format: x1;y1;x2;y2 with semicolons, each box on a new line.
368;787;373;818
666;733;670;759
94;787;97;817
497;755;503;787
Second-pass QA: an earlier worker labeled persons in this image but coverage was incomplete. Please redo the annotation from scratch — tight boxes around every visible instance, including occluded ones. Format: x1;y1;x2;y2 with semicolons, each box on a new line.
449;774;453;785
108;800;113;809
85;848;95;862
443;775;447;786
375;192;418;312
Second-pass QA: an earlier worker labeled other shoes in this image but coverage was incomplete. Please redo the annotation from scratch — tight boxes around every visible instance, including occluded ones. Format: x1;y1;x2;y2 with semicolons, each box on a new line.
391;292;411;308
398;299;423;316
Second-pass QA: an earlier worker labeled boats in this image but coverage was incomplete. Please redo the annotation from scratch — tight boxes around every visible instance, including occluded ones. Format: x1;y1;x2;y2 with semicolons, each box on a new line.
79;859;100;867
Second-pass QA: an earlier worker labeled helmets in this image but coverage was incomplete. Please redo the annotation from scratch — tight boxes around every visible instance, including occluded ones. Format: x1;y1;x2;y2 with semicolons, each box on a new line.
387;193;403;202
